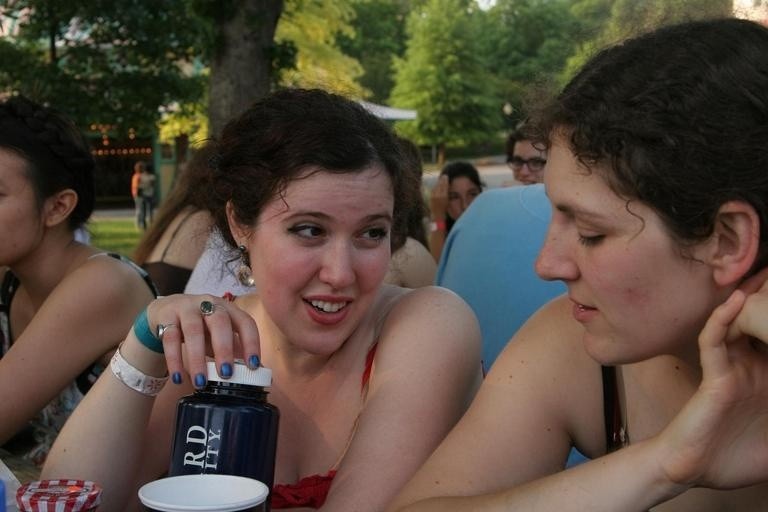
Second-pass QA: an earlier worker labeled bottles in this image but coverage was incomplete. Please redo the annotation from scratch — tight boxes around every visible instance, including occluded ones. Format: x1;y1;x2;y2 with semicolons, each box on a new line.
168;360;280;511
14;478;104;512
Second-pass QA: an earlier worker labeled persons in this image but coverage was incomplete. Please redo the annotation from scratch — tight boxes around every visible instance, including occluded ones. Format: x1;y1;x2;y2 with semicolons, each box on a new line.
379;17;767;511
435;183;589;468
0;98;160;467
384;137;439;288
132;161;155;229
506;126;549;185
432;162;482;262
36;87;484;511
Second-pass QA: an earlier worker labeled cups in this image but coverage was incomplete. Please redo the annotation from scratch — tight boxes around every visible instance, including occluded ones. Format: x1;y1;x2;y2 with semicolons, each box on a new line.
137;473;268;511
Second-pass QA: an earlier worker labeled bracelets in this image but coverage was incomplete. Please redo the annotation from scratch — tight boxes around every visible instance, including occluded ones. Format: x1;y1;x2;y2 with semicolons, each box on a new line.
132;303;164;354
432;221;446;231
110;342;169;397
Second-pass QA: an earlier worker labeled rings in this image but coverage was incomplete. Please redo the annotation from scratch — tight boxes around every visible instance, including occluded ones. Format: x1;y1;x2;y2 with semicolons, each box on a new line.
198;300;226;317
156;323;178;339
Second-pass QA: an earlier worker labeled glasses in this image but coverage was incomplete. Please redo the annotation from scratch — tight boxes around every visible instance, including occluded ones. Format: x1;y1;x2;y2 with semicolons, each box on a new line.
506;155;547;173
